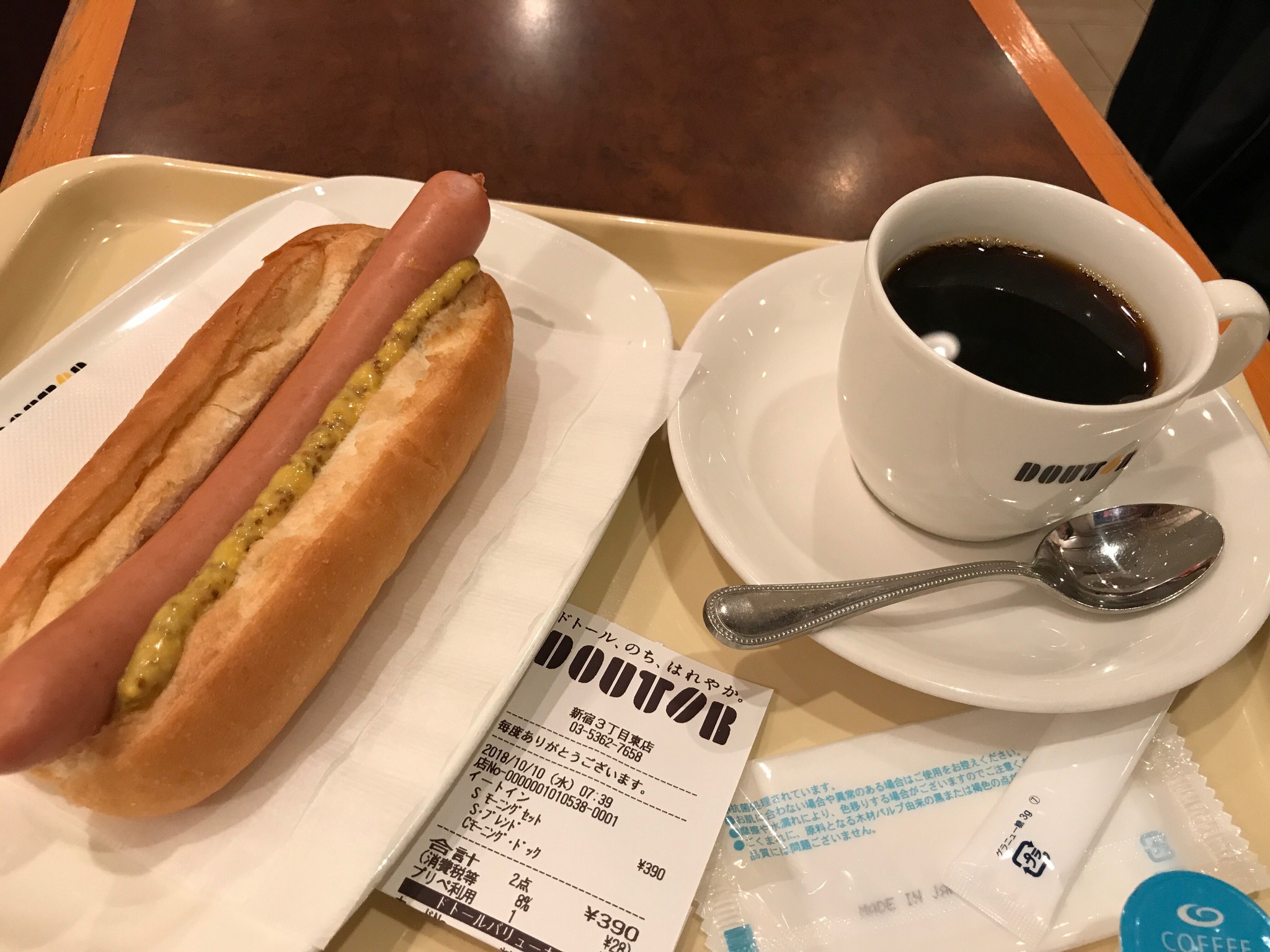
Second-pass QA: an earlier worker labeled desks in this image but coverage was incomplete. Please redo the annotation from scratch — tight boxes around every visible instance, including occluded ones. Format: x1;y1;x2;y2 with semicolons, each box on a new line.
0;0;1270;428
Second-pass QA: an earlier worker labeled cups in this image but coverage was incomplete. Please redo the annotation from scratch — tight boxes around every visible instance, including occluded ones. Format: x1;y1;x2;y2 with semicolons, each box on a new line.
839;175;1270;542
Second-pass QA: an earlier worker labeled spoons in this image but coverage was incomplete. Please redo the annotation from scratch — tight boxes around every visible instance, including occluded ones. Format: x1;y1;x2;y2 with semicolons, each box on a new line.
701;503;1224;650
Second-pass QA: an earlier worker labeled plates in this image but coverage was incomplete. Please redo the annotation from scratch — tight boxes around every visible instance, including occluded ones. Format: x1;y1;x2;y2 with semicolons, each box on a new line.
667;242;1270;713
1;176;674;952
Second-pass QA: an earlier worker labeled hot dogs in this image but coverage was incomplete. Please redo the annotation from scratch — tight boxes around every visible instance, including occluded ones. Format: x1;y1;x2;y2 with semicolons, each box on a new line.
0;168;515;819
